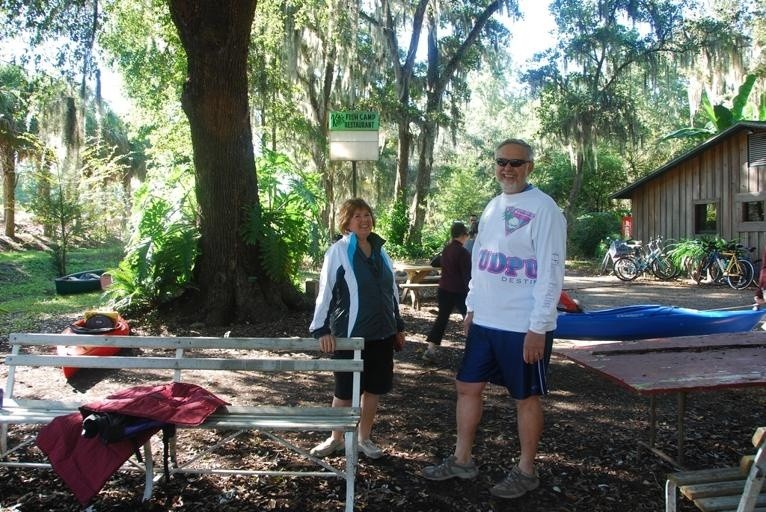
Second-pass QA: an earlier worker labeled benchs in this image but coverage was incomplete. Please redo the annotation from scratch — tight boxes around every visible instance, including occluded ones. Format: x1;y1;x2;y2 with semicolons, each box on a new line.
0;333;364;512
665;427;766;512
399;283;439;311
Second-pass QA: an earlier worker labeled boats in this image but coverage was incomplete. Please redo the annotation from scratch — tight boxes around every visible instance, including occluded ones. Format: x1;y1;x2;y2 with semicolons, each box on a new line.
54;268;106;295
57;311;131;379
554;304;766;343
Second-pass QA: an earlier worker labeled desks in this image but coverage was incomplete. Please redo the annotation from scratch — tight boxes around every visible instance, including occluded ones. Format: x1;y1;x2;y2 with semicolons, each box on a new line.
395;265;442;308
552;331;766;472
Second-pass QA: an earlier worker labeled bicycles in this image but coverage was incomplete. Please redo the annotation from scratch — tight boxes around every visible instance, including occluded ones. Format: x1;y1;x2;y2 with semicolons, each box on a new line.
612;235;763;291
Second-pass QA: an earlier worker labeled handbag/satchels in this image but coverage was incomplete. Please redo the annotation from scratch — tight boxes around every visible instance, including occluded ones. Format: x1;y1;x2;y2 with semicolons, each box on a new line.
430;255;441;267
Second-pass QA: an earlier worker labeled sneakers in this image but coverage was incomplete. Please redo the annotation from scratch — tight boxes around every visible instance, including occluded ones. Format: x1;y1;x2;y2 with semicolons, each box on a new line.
357;438;384;459
490;465;542;499
421;351;443;364
310;437;346;457
422;454;479;481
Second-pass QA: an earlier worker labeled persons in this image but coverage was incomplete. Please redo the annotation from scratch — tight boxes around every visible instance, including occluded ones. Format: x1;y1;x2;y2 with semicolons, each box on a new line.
464;219;480;254
422;217;479;363
414;138;567;497
470;214;478;225
752;248;766;311
308;195;407;461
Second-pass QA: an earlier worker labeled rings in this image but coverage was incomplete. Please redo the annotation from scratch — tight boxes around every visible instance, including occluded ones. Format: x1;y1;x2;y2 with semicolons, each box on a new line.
534;352;540;356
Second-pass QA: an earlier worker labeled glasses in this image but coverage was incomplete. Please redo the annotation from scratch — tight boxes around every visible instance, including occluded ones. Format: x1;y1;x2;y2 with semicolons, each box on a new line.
494;157;531;167
